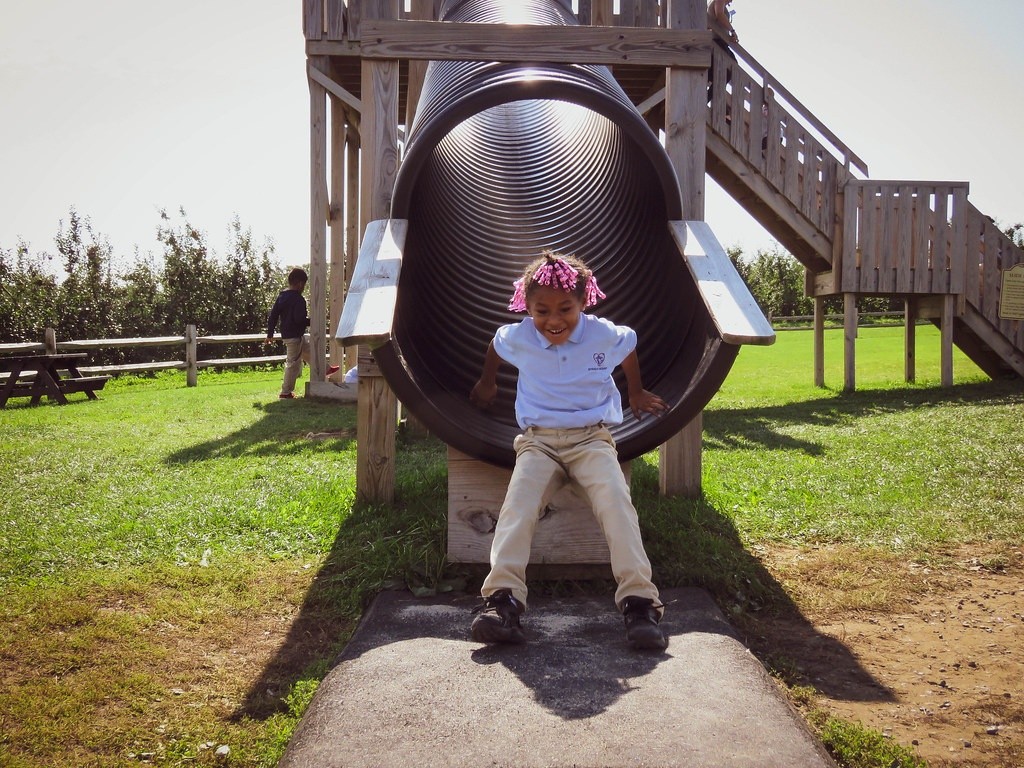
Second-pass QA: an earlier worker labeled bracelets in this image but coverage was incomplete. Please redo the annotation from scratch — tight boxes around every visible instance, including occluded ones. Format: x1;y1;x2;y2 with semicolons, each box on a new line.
730;28;735;33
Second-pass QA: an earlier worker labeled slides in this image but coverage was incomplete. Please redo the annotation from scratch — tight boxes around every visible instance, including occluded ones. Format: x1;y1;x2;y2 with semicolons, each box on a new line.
333;0;777;472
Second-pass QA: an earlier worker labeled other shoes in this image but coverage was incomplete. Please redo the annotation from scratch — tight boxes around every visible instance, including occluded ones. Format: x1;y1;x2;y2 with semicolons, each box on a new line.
323;366;340;379
279;393;296;400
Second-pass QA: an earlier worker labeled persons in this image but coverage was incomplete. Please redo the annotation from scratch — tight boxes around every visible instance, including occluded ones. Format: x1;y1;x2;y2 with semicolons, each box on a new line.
944;214;1020;334
470;252;674;651
706;0;739;104
762;87;783;159
265;268;340;400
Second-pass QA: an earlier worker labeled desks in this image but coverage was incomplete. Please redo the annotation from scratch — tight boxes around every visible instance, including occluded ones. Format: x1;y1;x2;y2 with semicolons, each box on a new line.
0;352;99;409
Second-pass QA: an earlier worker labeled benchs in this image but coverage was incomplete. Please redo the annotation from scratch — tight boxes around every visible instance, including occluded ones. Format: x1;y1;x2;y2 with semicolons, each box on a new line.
0;382;35;395
59;376;113;393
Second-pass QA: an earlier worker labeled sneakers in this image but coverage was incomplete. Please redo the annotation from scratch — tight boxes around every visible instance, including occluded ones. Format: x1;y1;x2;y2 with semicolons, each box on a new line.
469;589;527;645
622;596;671;648
